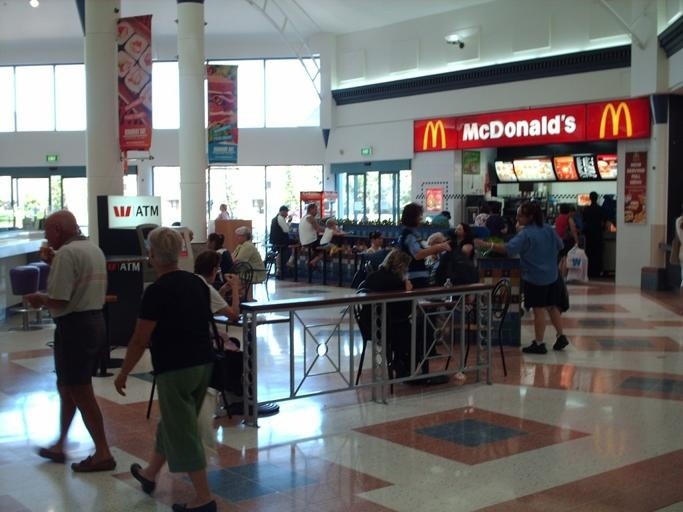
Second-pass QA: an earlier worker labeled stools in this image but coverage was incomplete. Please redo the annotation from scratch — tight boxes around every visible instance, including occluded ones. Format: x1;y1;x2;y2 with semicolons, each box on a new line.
27;262;49;323
277;244;358;287
10;266;39;331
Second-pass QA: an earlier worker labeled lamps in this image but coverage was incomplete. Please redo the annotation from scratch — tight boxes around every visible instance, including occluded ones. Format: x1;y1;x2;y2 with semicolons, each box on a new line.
444;33;464;49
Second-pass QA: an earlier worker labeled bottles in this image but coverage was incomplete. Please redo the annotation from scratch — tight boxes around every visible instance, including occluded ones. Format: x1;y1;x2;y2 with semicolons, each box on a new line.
444;279;452;303
180;232;188;256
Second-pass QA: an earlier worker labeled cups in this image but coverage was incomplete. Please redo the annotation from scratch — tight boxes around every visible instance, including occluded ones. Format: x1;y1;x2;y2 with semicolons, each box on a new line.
40;246;48;261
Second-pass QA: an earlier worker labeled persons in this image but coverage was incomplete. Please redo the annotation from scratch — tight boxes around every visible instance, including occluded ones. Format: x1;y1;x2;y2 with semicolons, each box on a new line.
24;211;114;472
208;234;238;295
114;226;217;511
270;206;301;268
231;227;266;284
556;193;616;279
298;203;324;268
192;252;244;415
319;217;347;262
366;200;569;378
216;204;230;220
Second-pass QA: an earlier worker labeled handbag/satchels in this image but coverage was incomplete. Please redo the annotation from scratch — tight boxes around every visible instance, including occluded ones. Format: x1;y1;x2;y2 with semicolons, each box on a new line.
205;349;225;391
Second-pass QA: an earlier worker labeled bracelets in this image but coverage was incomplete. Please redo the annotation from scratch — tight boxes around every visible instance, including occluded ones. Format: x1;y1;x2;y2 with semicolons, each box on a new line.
120;371;128;377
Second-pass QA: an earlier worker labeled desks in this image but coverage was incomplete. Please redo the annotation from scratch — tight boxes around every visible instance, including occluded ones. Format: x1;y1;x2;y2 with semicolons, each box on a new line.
212;313;290;334
418;301;456;309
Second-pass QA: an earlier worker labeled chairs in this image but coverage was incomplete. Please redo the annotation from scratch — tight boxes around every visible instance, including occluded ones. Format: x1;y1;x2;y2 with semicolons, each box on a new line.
446;277;511;376
147;247;280;422
354;287;412;395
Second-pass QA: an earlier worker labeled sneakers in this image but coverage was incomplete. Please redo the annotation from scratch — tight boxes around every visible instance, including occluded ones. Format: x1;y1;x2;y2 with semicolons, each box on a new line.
171;499;219;511
522;341;547;355
39;448;66;463
130;462;156;493
72;456;117;472
552;336;569;350
